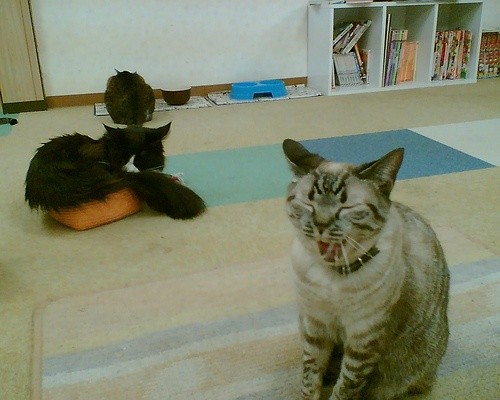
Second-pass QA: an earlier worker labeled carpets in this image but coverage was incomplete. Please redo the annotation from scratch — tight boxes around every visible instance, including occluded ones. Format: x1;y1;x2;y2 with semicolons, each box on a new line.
30;212;500;399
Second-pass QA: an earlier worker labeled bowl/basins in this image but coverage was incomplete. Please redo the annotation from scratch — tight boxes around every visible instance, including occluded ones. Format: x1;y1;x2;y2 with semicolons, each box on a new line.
161;86;192;106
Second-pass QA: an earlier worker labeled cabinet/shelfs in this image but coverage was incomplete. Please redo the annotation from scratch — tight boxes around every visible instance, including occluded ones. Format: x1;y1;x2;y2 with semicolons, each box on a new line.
309;1;485;96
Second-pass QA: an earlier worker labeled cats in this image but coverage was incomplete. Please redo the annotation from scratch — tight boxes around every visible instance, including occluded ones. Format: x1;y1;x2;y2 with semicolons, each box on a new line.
103;68;156;127
280;137;452;397
23;121;208;222
